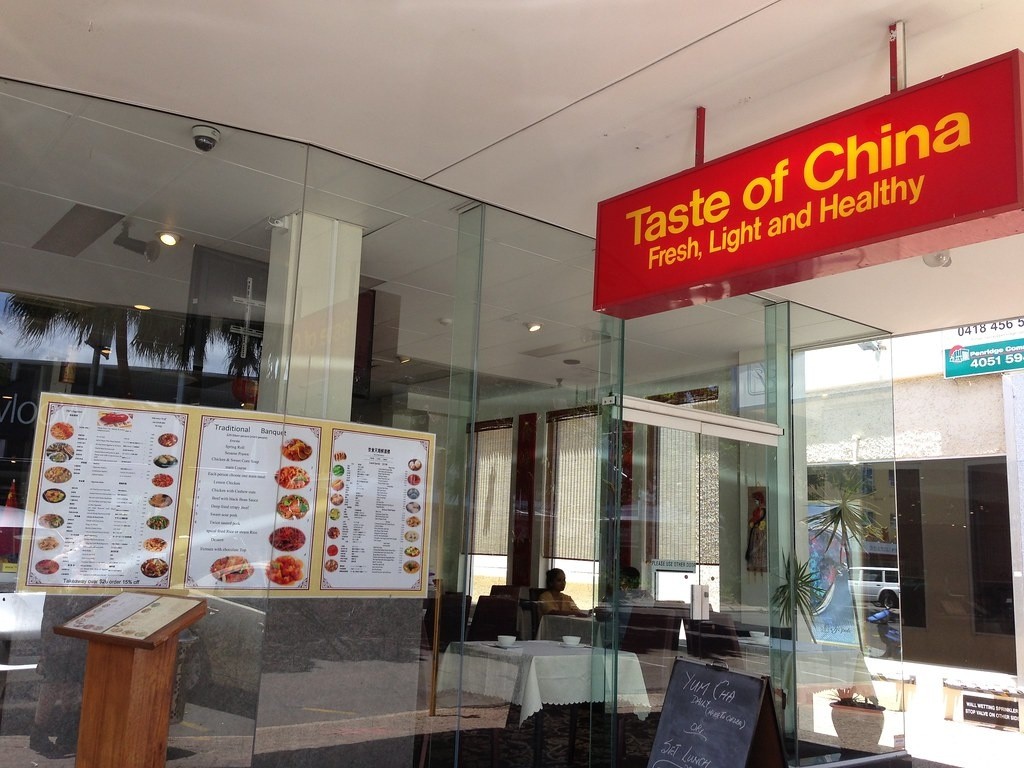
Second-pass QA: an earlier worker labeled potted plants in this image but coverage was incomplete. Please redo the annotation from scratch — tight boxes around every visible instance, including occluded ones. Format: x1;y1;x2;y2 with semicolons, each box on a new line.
774;493;895;745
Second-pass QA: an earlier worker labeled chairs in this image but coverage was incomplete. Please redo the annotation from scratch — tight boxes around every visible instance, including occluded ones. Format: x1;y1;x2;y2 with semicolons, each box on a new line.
423;577;746;655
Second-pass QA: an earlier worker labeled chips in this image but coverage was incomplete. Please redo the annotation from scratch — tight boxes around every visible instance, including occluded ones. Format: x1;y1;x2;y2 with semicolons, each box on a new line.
283;439;311;460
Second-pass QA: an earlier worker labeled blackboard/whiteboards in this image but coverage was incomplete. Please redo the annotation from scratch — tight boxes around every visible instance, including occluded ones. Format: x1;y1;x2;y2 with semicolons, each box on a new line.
646;656;788;768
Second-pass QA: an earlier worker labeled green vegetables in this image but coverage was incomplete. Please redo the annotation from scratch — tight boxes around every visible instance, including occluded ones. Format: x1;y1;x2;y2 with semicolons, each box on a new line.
151;516;167;530
334;466;344;476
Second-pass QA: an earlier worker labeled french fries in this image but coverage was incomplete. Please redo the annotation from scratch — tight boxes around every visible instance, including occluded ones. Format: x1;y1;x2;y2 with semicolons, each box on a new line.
280;468;306;488
150;559;167;577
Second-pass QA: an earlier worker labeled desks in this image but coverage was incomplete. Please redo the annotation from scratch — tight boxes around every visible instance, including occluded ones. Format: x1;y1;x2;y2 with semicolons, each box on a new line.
437;642;649;767
536;613;621;643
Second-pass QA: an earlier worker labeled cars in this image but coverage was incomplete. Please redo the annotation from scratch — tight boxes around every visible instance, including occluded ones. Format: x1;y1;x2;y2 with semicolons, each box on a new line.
182;596;265;697
0;506;47;710
848;567;900;608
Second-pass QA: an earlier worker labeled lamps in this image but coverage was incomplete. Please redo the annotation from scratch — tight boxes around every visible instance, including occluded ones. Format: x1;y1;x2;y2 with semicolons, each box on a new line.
399;355;410;364
156;230;185;245
527;322;542;333
102;346;113;353
113;225;161;265
923;248;953;270
859;339;886;352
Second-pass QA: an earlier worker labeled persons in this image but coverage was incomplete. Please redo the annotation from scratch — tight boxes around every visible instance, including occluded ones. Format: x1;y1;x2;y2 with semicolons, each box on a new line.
601;566;655;649
536;568;589;621
